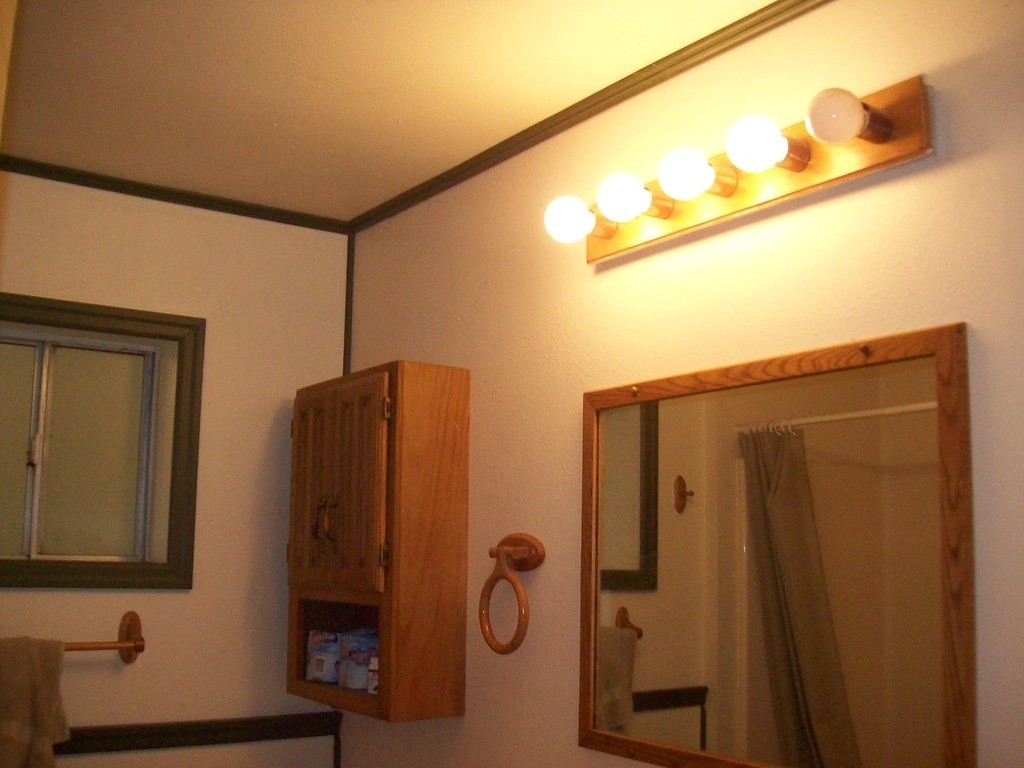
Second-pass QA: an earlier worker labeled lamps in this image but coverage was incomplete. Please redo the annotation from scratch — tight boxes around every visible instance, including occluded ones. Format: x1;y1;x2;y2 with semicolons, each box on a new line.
543;85;887;245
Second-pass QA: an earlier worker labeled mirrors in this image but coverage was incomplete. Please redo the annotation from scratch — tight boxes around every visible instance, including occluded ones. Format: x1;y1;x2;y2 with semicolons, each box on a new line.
578;320;976;768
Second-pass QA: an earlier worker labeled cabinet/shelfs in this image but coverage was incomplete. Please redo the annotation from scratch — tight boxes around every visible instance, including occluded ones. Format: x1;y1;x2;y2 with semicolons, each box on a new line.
286;358;472;723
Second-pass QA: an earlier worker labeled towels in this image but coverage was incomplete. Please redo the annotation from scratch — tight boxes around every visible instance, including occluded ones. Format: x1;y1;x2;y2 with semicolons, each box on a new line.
0;636;71;768
596;625;638;733
